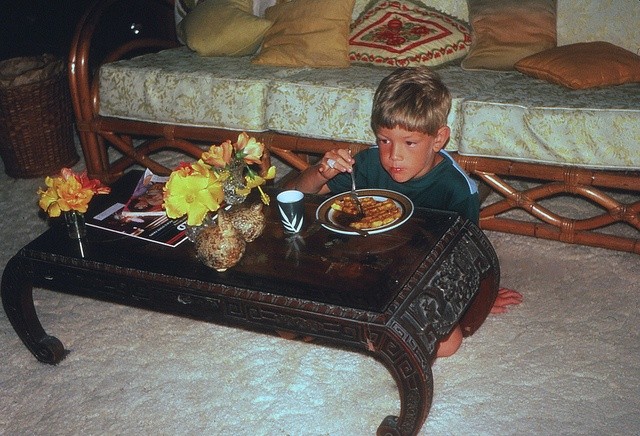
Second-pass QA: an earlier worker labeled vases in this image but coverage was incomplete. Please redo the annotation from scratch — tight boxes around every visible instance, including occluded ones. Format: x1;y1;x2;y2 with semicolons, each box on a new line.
185;212;216;242
223;165;248;205
64;211;87;240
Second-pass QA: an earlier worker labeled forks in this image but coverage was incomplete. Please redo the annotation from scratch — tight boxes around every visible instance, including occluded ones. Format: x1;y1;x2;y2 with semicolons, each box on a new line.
349;150;363;222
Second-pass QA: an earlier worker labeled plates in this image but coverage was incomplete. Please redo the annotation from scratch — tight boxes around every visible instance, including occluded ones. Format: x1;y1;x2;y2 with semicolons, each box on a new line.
316;189;414;236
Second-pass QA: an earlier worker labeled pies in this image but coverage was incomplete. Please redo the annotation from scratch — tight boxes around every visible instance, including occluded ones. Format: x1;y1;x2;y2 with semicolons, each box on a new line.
333;197;403;230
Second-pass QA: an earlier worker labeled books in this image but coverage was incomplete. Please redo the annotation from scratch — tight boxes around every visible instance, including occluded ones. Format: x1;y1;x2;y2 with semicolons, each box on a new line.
84;200;231;248
122;168;171;217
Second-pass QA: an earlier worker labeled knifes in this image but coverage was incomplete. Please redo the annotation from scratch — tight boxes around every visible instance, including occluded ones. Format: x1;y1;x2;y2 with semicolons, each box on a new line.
314;219;369;238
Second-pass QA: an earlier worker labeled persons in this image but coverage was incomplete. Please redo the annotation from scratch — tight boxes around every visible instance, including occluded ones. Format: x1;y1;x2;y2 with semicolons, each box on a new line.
127;168;164;212
293;65;524;358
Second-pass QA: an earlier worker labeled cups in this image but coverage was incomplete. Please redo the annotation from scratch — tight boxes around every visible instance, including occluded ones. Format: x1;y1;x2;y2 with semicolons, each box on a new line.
276;190;304;235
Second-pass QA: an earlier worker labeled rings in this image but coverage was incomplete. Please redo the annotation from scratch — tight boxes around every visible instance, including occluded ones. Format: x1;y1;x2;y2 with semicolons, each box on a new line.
325;158;336;169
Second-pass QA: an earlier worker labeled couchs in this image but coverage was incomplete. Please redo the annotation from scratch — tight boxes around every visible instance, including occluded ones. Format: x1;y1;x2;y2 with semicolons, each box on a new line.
67;0;640;253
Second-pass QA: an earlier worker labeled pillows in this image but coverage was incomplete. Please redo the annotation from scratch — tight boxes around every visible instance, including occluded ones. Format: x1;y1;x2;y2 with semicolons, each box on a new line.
461;0;558;71
513;42;640;89
176;0;276;57
251;0;356;68
349;0;472;68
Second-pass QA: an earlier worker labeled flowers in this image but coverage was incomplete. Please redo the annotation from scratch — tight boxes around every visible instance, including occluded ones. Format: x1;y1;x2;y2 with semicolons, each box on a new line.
38;168;111;218
160;158;231;227
202;131;276;205
